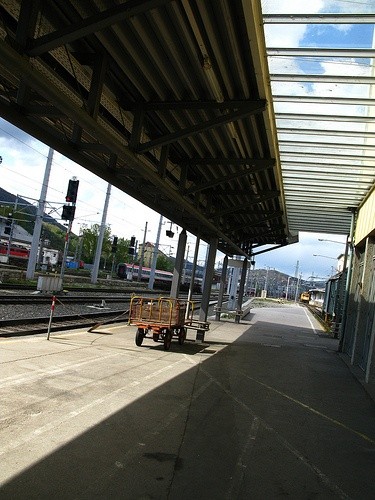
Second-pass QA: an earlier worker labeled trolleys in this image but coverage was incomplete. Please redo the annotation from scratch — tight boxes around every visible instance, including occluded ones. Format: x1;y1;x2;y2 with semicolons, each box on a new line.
127;296;194;351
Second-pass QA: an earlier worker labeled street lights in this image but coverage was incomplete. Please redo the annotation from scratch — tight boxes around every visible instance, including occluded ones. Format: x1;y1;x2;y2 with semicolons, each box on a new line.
286;276;291;300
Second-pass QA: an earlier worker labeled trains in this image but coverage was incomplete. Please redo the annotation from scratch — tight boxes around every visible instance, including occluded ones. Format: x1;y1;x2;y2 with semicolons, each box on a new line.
0;238;59;271
116;262;203;293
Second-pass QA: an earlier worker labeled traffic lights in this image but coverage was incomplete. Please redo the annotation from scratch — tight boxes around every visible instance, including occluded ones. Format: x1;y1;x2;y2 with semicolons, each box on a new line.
65;179;80;203
